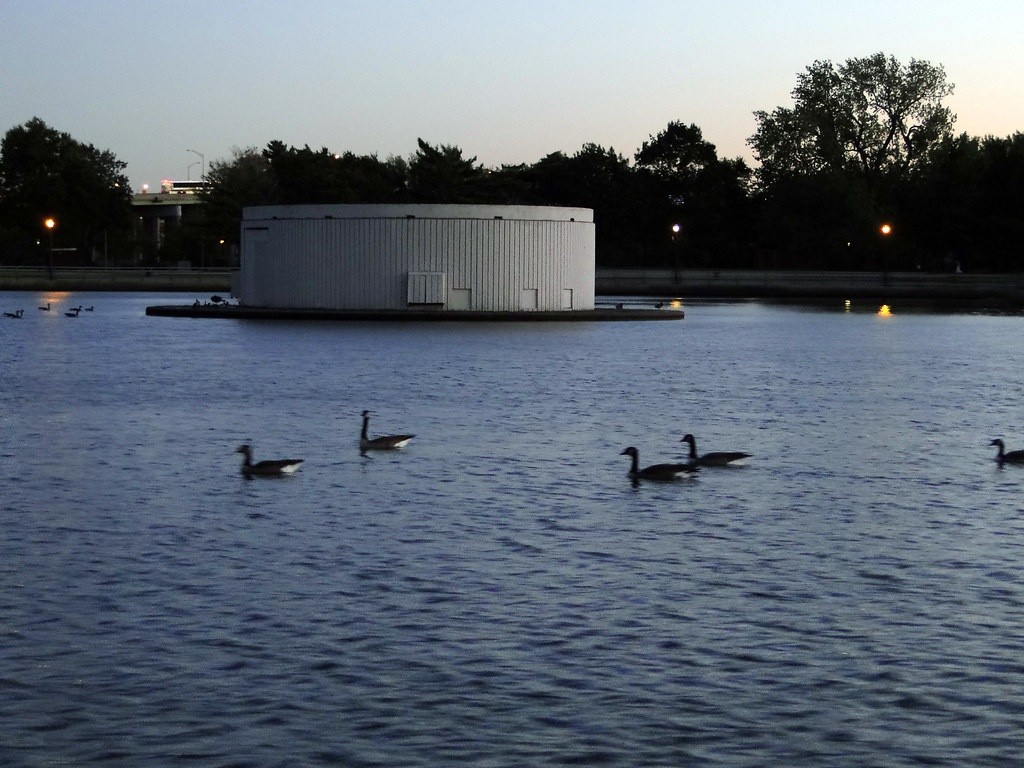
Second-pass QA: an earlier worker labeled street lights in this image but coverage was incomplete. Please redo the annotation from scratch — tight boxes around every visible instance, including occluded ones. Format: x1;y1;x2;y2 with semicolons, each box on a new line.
45;218;54;281
673;223;680;285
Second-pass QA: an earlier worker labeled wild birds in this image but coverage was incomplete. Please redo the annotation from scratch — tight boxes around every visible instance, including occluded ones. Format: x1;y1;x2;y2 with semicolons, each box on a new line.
235;444;305;479
360;409;419;449
3;302;95;320
679;433;755;466
654;302;664;310
192;295;229;306
988;439;1024;465
619;446;692;481
